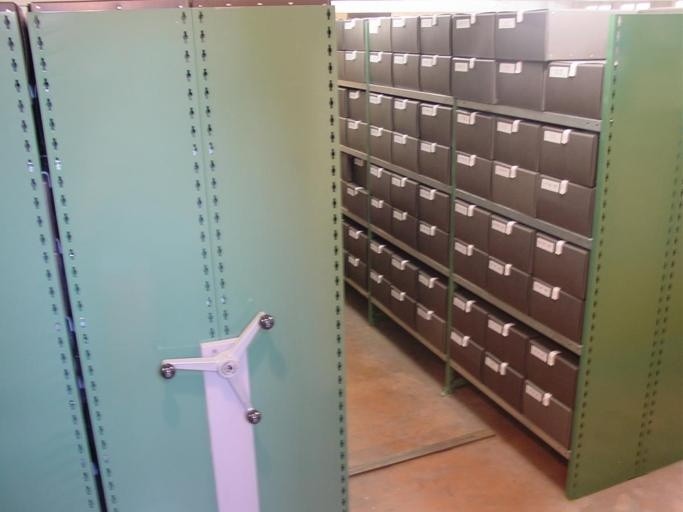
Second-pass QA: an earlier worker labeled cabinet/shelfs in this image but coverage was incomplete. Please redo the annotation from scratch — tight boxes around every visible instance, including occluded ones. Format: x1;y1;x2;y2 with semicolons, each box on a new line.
449;9;683;502
2;4;353;512
336;4;449;395
